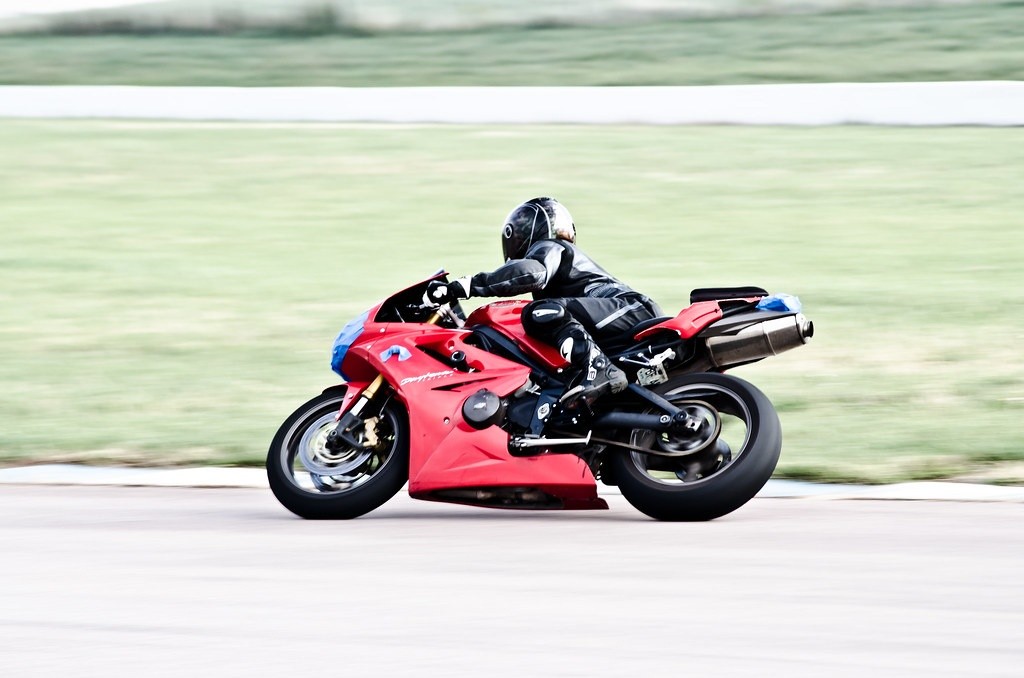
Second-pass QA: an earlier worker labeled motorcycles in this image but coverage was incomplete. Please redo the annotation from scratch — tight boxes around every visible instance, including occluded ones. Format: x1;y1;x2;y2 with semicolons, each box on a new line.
265;268;815;523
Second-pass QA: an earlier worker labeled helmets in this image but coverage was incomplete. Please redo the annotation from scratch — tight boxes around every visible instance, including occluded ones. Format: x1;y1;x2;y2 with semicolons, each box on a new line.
502;196;576;261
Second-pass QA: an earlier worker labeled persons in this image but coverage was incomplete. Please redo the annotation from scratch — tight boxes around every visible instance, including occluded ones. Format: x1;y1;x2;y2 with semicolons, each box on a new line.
428;197;660;408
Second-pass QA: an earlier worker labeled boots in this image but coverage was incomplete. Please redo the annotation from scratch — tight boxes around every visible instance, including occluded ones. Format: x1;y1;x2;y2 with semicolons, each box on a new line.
554;319;628;412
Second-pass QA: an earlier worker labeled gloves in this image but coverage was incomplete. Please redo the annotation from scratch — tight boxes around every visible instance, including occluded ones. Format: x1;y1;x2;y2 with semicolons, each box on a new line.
419;274;472;311
